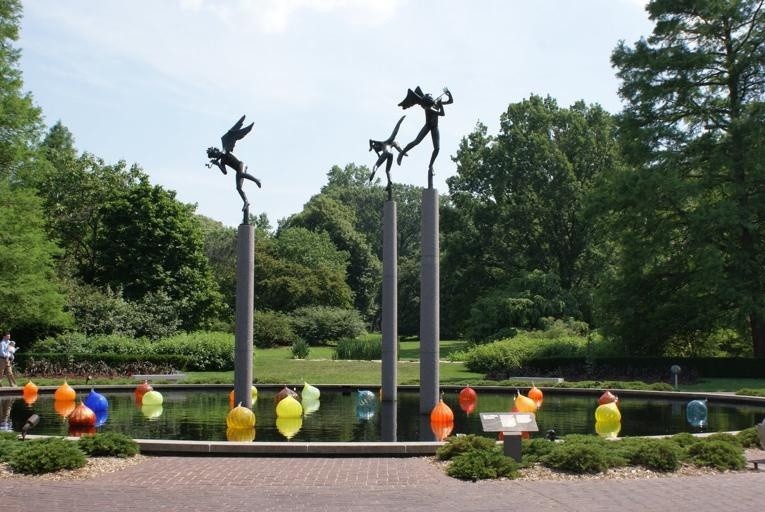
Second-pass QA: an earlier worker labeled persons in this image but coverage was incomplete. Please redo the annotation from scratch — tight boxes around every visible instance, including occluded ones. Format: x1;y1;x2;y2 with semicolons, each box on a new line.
397;88;453;171
0;332;16;388
370;112;406;190
206;114;261;212
5;340;19;366
